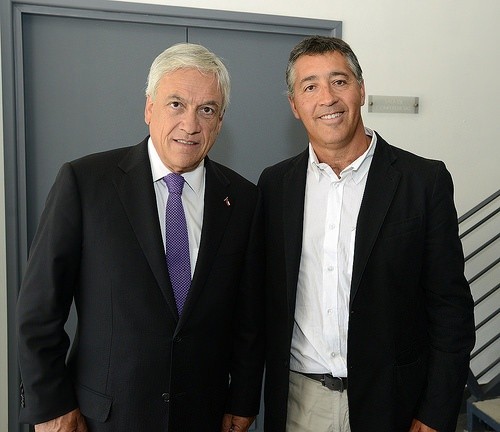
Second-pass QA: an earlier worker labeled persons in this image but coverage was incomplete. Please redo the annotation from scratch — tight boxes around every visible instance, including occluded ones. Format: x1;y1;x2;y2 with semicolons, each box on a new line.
256;36;476;432
17;44;260;431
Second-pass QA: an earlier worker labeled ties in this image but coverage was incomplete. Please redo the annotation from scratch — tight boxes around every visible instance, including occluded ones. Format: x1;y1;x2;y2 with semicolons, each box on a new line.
163;172;191;318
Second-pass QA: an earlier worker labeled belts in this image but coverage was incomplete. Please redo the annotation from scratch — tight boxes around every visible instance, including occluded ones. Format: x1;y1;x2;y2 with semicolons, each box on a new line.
290;370;347;393
